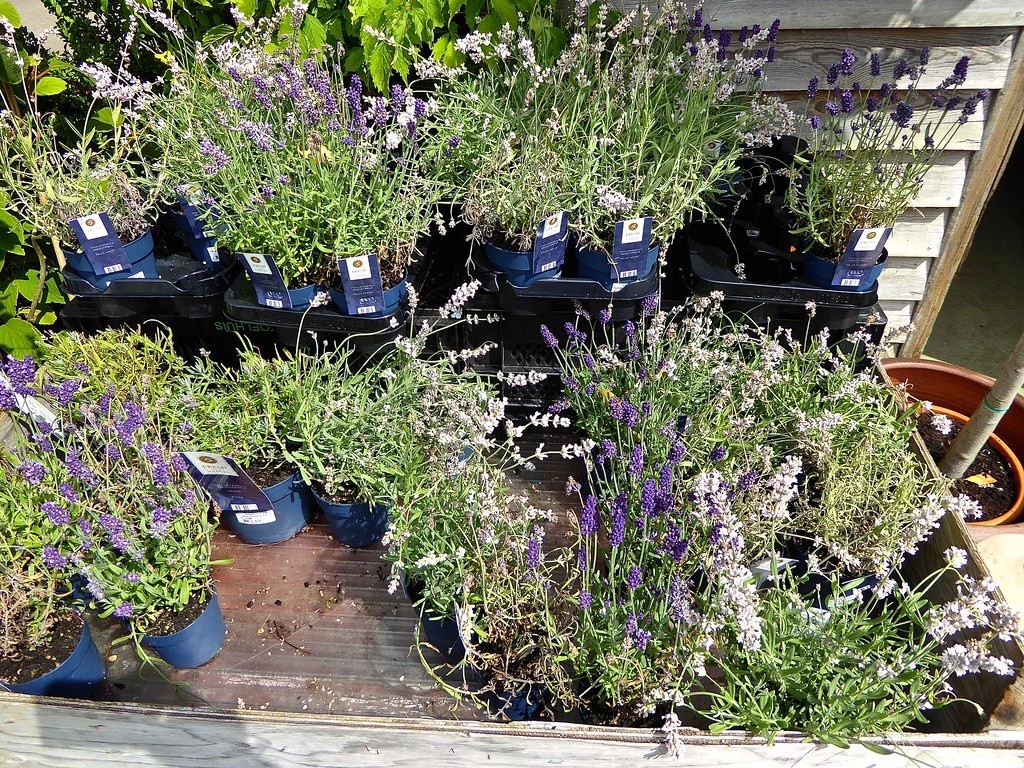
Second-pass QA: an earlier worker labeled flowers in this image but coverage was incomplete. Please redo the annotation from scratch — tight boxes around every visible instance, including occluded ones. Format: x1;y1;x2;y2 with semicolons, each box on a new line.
0;0;1024;757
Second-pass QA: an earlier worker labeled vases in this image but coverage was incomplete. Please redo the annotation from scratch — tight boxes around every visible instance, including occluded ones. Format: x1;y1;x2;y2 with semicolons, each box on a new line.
59;227;161;292
58;570;96;604
171;200;233;274
583;248;658;286
482;230;568;287
408;571;475;652
254;281;317;311
324;263;408;316
310;481;390;545
802;245;888;291
1;614;107;699
499;682;547;722
134;580;227;671
221;465;316;545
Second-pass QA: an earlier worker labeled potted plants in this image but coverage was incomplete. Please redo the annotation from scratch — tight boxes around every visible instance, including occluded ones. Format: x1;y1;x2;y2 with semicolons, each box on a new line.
908;336;1024;529
